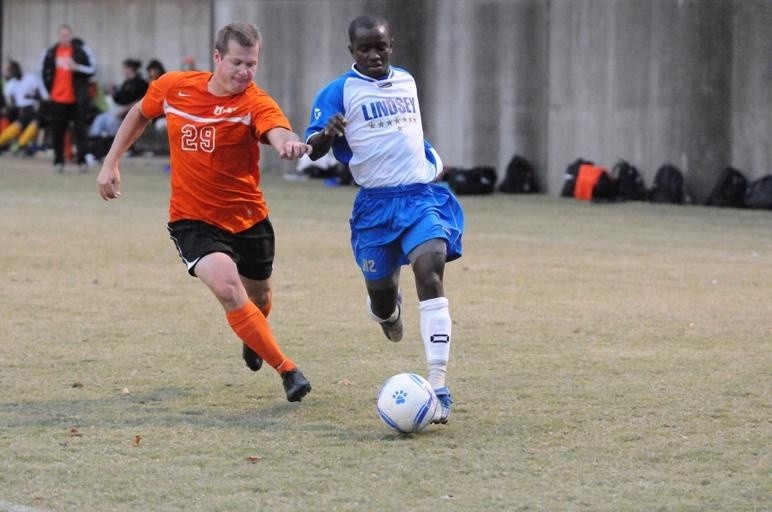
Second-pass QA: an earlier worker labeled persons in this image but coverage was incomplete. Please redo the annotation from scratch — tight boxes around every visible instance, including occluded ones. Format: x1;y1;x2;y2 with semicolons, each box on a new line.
306;15;465;422
0;22;196;175
94;22;313;404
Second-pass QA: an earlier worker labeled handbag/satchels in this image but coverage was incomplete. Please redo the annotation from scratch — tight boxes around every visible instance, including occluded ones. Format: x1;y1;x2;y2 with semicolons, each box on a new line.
442;167;496;194
561;160;684;205
499;155;538;193
703;165;771;208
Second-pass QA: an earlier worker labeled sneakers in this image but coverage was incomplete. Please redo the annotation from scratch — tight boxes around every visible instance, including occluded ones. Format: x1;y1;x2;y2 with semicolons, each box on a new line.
281;368;311;402
54;162;62;172
79;163;89;174
434;387;454;424
243;342;263;371
379;287;402;344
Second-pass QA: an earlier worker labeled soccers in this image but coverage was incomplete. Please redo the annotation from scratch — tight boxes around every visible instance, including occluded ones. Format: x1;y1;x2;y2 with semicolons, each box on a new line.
376;373;438;434
